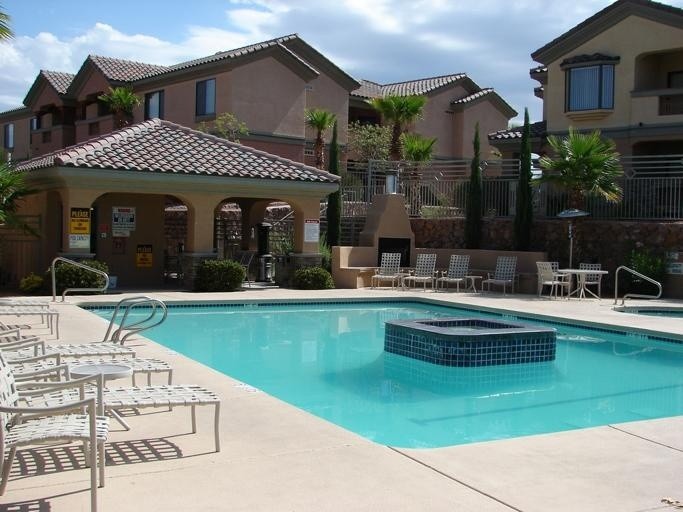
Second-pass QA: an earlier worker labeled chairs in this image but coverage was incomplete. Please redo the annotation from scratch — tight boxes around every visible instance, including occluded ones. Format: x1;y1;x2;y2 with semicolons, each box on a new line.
159;255;184;289
372;252;609;300
221;251;255;286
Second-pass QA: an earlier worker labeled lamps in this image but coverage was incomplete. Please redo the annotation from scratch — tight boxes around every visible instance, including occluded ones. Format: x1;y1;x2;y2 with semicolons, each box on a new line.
556;207;591;270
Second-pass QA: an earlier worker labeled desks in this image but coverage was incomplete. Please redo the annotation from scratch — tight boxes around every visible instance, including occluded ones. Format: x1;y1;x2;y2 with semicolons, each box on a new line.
181;252;217;290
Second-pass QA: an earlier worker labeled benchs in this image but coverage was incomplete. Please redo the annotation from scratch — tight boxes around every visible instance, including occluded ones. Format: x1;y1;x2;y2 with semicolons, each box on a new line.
330;245;548;289
